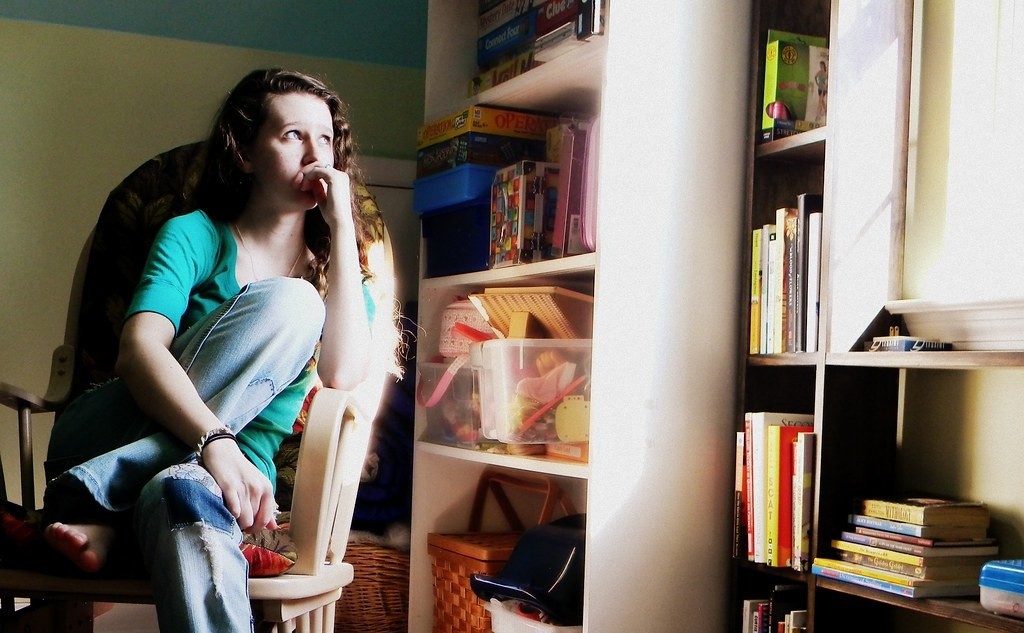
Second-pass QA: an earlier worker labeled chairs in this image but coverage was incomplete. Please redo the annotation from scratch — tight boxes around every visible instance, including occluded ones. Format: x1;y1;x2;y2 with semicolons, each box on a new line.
0;212;365;633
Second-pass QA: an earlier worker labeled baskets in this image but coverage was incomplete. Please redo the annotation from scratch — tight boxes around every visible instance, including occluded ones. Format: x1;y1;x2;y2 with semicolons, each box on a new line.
425;465;578;633
277;524;411;633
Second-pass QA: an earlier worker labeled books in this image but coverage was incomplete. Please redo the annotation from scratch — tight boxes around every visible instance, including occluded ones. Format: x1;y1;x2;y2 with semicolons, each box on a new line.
812;496;998;599
743;585;807;633
732;412;813;571
750;193;821;353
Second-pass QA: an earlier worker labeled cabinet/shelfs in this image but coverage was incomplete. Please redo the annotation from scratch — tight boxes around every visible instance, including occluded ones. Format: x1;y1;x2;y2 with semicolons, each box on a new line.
409;0;1024;633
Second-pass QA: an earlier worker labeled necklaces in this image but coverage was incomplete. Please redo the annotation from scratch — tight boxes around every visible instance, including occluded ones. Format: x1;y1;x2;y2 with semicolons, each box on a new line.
235;224;306;281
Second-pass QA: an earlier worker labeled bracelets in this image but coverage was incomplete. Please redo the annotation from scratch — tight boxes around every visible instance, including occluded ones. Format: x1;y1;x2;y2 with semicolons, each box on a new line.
201;433;239;450
196;427;231;460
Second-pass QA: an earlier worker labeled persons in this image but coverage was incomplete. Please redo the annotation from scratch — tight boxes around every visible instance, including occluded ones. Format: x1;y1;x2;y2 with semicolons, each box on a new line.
815;62;828;120
42;70;391;633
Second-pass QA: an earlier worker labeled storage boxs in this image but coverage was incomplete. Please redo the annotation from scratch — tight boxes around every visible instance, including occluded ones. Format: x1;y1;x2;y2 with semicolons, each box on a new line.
413;360;504;448
413;105;600;276
762;28;830;139
481;599;584;633
470;337;592;448
978;559;1024;618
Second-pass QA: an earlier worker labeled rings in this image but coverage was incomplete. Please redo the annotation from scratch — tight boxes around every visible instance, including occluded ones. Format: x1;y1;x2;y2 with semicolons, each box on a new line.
325;164;331;168
